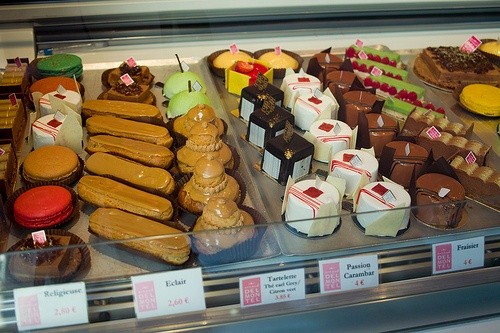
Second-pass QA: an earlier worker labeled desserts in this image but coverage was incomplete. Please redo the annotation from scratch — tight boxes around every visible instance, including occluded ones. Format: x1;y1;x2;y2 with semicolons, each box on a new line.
207;38;500;237
0;52;267;285
